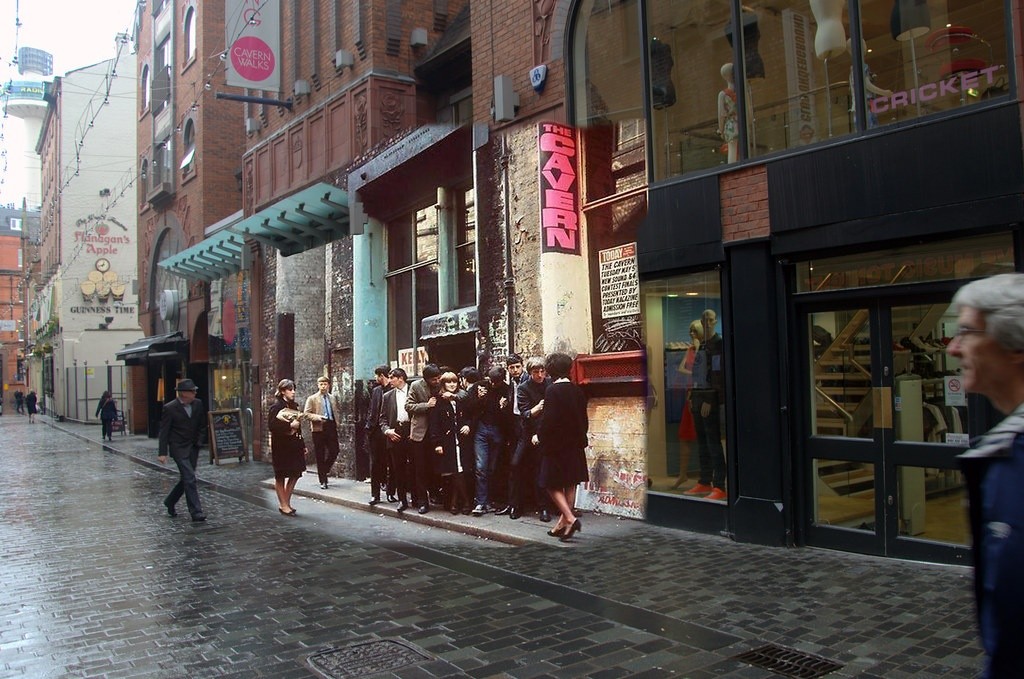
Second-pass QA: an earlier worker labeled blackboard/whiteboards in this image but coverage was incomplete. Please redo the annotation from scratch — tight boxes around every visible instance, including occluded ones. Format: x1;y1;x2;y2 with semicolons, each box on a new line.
208;408;246;459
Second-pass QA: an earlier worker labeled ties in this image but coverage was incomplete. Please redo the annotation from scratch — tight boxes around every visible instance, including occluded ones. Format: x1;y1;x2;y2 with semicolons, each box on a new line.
323;394;330;420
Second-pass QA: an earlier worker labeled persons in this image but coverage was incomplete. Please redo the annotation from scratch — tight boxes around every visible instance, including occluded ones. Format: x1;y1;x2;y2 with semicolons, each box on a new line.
810;0;848;62
158;379;207;521
944;271;1023;679
14;388;24;413
846;37;894;132
724;6;766;80
304;376;341;489
648;36;676;111
26;390;37;424
799;124;814;145
365;353;591;542
717;63;741;165
267;379;309;516
675;310;726;500
890;0;931;42
96;391;118;440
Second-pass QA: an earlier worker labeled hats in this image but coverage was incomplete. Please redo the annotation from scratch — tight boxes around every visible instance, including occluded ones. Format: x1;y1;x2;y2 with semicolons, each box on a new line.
175;379;199;391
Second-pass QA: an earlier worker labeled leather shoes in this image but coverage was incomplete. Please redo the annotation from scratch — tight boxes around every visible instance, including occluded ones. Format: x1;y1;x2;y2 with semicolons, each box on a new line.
539;506;551;522
510;505;522;518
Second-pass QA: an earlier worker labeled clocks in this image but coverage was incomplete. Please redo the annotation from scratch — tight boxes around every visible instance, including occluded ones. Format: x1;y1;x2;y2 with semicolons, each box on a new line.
96;258;110;272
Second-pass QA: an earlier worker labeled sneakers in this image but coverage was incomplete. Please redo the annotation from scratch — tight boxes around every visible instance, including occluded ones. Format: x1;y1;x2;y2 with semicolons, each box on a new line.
703;488;727;500
683;484;712;495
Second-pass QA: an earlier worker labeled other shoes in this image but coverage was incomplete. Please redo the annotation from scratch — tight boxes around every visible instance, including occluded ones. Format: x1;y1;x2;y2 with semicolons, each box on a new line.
387;494;397;502
368;496;380;505
397;502;511;515
321;481;328;490
571;507;582;517
192;512;207;520
547;519;581;542
163;502;177;516
279;506;297;515
